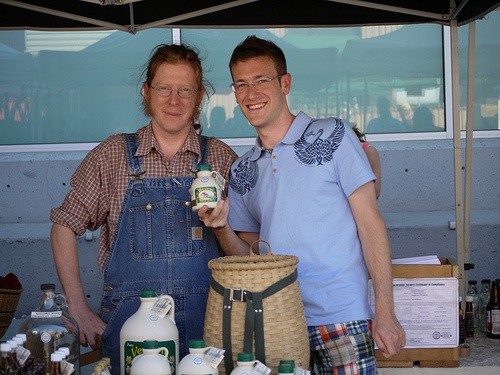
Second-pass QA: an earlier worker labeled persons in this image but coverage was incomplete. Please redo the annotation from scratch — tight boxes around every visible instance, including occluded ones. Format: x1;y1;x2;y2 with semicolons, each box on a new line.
49;42;244;375
194;97;445;138
195;34;405;375
347;126;383;201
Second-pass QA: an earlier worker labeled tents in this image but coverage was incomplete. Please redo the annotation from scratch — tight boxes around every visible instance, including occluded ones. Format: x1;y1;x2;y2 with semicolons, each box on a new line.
0;1;500;323
0;24;500;131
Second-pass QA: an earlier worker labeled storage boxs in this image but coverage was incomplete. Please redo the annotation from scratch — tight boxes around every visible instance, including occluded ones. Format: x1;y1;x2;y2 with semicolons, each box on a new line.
368;258;461;369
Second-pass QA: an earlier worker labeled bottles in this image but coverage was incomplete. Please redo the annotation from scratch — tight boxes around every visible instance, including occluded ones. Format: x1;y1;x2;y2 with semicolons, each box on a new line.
19;283;81;375
121;290;180;375
1;333;29;375
130;339;171;375
466;278;479;328
464;295;475;338
90;357;113;375
192;163;223;211
485;281;500;339
477;279;492;332
457;295;466;345
178;338;220;375
229;354;267;375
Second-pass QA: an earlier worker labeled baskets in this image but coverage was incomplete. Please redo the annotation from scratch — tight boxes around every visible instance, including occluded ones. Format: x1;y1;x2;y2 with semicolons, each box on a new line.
202;240;308;375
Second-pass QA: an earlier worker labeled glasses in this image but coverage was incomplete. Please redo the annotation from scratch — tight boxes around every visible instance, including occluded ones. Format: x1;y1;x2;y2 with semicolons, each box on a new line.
147;81;205;98
228;71;288;93
